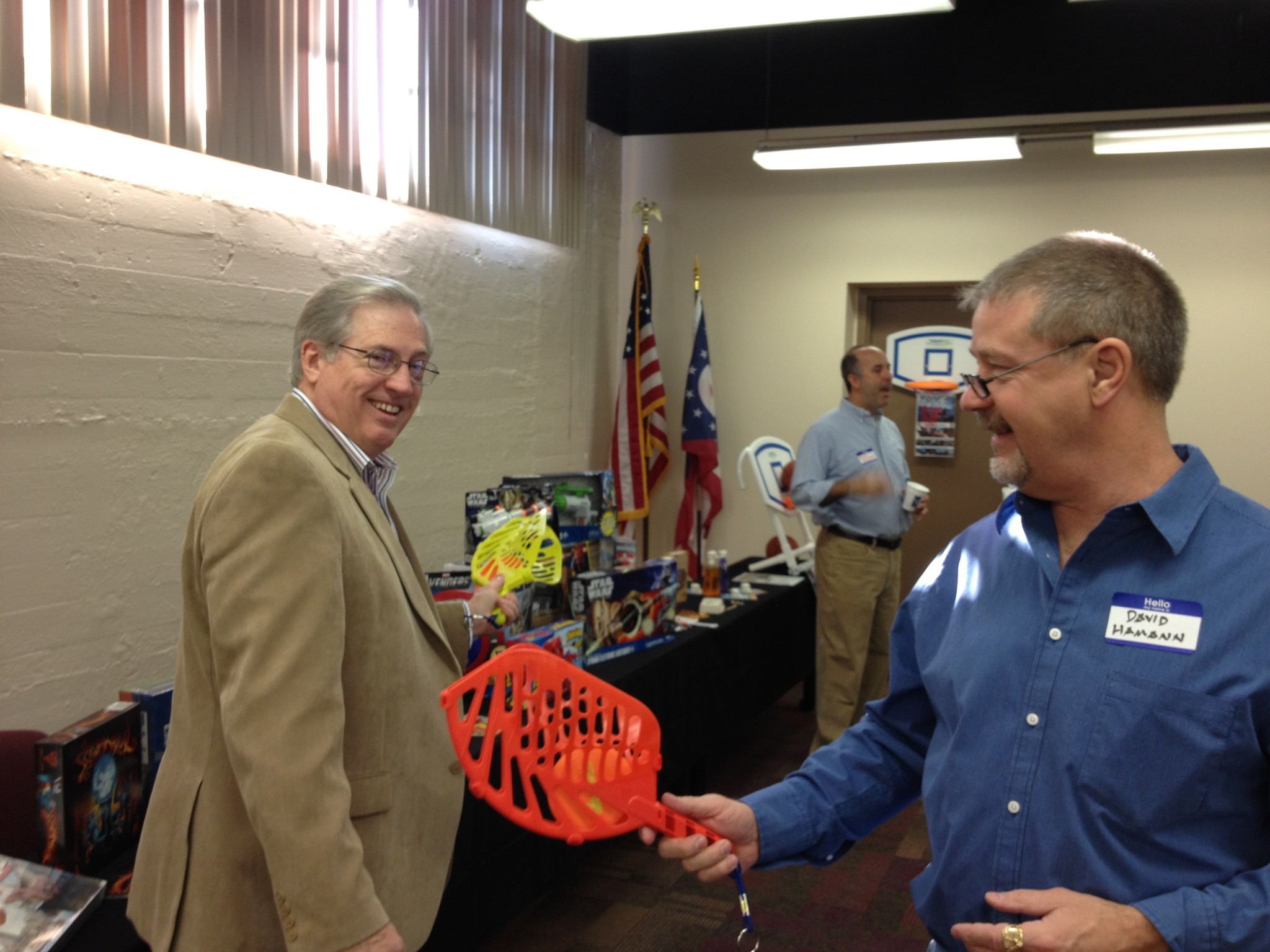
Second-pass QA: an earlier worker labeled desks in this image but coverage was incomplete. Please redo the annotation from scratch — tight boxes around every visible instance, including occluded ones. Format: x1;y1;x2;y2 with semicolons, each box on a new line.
443;558;816;902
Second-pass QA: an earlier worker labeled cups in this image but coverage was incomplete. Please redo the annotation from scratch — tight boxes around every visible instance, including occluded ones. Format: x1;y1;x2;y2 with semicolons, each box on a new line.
902;481;930;513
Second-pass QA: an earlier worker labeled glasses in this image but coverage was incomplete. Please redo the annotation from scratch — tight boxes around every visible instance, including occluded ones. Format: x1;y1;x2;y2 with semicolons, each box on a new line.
960;338;1100;399
326;341;441;386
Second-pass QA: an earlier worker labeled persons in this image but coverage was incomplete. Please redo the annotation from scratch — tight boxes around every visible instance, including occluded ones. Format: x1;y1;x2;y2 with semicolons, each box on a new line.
640;231;1269;952
791;345;932;758
125;273;476;952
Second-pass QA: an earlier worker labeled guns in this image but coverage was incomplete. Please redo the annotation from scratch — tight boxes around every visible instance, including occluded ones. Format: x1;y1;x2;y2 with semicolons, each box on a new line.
471;501;552;539
553;482;598;526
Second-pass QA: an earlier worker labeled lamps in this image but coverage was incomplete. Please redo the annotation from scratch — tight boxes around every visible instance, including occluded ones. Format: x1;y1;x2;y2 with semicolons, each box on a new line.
1091;115;1270;156
753;123;1022;172
523;1;958;45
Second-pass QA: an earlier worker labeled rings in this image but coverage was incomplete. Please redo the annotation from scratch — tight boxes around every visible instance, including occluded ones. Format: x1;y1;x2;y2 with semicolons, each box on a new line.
1002;923;1024;952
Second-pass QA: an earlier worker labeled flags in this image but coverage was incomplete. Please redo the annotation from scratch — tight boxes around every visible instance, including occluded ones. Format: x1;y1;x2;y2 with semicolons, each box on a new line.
675;290;723;583
602;230;672;573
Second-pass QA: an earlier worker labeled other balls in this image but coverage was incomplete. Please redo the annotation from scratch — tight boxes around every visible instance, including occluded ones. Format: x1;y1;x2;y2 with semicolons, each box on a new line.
782;461;796;490
767;536;798;557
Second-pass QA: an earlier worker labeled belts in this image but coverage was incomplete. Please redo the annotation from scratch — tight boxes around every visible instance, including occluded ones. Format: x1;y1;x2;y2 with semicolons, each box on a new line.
826;524;903;551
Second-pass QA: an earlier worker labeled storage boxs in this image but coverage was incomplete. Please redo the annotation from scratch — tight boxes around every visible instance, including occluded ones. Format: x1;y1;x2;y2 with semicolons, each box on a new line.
0;679;173;952
426;471;679;707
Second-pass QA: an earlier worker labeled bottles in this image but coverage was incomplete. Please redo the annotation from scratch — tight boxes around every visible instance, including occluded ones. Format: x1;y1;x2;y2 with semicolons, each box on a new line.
719;551;729;592
703;550;721;597
